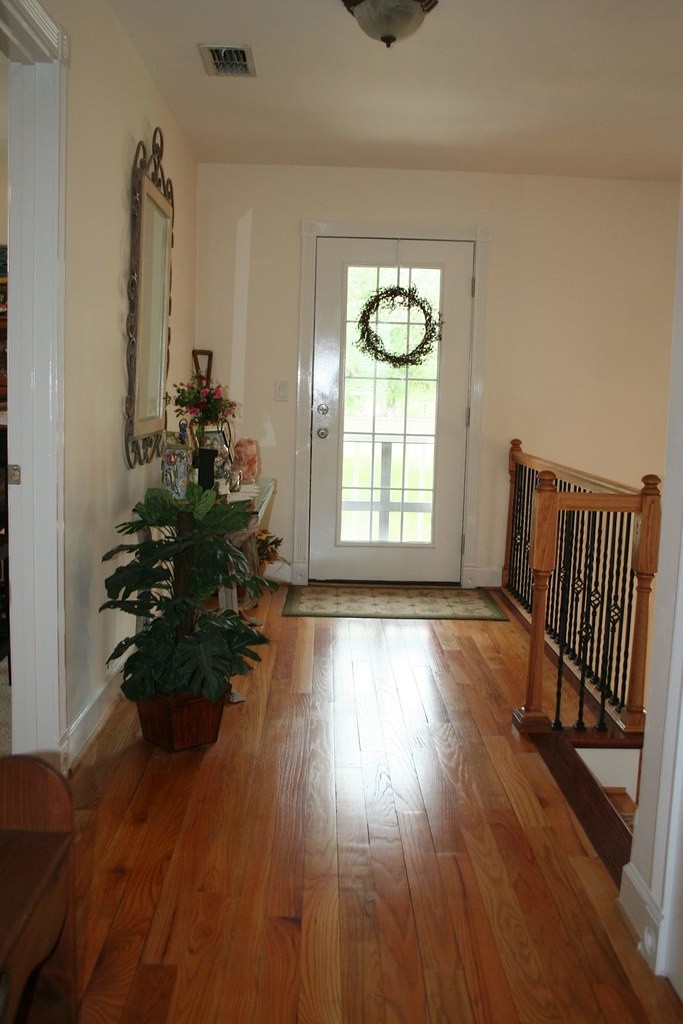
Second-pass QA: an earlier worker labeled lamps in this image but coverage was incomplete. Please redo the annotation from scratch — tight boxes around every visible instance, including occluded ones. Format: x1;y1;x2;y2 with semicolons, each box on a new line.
341;0;439;48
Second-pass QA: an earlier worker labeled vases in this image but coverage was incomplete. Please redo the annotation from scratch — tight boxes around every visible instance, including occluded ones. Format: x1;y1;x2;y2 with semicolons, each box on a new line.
196;422;211;447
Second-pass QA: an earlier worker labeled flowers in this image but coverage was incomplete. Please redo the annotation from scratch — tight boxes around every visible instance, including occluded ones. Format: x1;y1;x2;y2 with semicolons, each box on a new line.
174;367;237;422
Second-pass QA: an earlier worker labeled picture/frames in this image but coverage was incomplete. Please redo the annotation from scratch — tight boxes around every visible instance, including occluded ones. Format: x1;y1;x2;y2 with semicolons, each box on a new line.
200;430;234;468
124;126;175;471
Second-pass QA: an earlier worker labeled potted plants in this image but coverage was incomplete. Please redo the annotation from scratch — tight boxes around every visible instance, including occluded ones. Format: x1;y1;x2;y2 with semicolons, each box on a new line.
97;480;281;751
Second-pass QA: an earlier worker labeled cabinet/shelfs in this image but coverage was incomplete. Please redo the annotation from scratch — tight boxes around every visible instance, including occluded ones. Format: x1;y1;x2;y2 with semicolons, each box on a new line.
0;748;80;1024
216;480;274;614
